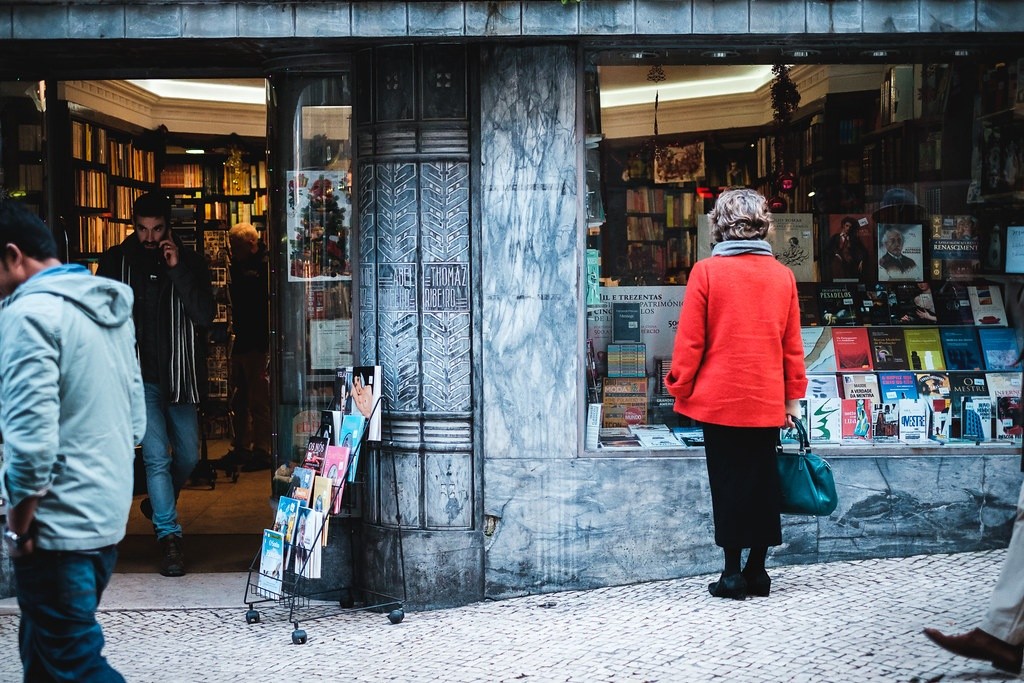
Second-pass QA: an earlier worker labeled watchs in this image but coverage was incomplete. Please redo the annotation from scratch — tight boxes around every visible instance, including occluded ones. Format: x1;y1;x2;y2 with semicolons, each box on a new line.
3;526;31;547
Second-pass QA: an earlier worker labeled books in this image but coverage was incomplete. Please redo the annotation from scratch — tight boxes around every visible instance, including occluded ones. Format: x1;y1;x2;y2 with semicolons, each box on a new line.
586;159;1023;447
72;116;280;438
256;364;381;601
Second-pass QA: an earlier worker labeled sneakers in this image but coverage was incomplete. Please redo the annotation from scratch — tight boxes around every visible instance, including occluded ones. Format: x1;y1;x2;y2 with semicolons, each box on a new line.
140;498;155;521
158;535;185;576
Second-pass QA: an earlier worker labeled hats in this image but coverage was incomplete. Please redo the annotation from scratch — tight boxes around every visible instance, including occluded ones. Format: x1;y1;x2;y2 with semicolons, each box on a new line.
872;188;926;222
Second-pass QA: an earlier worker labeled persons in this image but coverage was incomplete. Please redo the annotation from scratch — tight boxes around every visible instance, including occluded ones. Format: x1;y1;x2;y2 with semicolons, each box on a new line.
823;308;834;325
878;229;917;273
920;479;1023;675
823;218;869;280
0;196;149;683
664;190;809;600
219;221;273;473
900;283;937;324
94;193;217;578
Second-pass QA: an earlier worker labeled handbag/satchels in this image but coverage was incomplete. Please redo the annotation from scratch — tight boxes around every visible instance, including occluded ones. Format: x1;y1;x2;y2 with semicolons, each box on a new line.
774;414;838;516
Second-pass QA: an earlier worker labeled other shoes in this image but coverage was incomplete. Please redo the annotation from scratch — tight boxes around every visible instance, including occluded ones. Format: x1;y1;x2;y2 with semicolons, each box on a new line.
742;566;771;597
241;448;269;472
708;571;748;601
218;447;251;467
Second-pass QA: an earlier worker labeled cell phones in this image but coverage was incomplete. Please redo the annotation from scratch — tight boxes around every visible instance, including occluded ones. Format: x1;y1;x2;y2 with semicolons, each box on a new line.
164;232;170;259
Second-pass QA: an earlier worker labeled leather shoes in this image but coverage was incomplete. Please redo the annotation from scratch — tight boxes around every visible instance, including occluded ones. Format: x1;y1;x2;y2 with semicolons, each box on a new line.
924;626;1023;675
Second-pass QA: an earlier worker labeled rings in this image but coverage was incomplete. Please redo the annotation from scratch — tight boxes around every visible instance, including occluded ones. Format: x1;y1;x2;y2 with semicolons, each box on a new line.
166;245;170;249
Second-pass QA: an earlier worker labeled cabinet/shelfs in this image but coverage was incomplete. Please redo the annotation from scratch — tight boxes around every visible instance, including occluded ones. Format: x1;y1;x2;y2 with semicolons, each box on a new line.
597;58;1024;284
0;96;267;437
